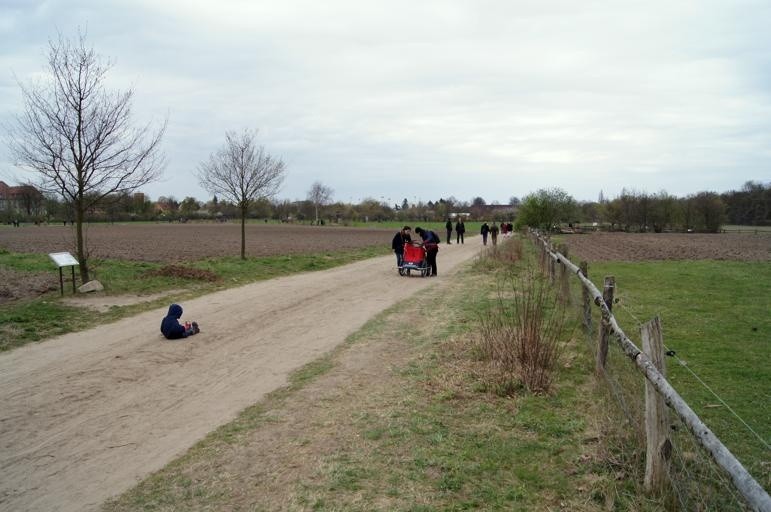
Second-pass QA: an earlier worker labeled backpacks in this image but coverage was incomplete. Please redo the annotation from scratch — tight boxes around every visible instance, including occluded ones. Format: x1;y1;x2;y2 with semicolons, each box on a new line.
430;230;440;243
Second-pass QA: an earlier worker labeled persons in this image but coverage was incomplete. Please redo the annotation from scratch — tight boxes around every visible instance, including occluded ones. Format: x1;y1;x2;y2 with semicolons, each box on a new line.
161;304;200;340
445;217;465;244
481;219;513;246
392;226;438;277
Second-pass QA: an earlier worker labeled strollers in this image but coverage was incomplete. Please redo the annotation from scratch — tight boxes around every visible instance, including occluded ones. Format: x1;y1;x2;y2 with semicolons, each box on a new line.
396;240;428;277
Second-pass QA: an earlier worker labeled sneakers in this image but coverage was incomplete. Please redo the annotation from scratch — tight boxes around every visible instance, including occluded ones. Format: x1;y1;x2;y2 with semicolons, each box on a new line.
184;321;200;333
426;273;437;277
